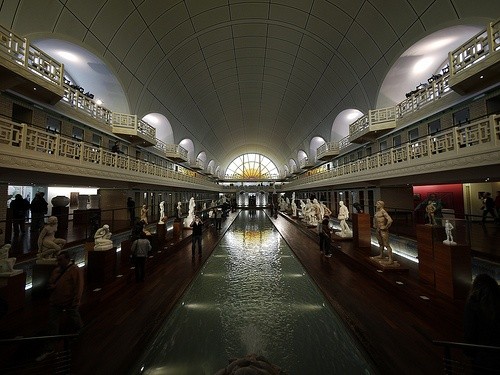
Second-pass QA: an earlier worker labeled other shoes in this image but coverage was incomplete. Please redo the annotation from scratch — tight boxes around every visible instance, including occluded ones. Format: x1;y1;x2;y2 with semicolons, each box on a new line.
320;251;324;254
325;254;333;257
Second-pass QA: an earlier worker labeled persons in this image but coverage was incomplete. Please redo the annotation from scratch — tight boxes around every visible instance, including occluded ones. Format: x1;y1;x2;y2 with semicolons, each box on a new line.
319;215;333;258
44;249;84;343
426;201;437;225
130;232;152;284
9;190;48;237
270;205;278;218
444;220;454;242
459;271;500;375
0;195;200;274
480;190;500;232
372;199;395;266
189;215;204;257
276;196;352;237
216;209;224;230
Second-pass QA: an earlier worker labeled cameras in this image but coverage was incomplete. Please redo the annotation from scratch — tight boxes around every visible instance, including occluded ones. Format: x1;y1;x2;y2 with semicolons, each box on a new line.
195;218;199;221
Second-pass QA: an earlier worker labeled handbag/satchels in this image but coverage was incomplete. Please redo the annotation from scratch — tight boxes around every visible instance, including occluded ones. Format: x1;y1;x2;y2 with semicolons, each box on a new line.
128;240;138;263
32;284;56;309
